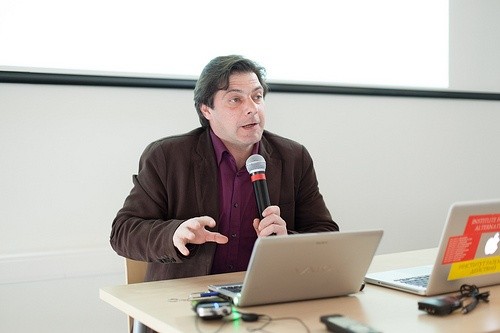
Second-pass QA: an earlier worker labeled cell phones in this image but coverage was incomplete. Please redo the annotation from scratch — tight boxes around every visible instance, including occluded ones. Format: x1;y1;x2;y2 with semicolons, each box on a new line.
321;314;384;333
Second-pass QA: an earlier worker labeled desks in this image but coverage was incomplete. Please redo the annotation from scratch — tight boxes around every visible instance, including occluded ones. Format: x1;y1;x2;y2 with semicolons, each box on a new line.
98;248;500;333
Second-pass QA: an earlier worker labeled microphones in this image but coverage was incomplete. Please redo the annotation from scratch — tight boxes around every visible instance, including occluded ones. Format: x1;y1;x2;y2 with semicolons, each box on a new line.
246;154;276;236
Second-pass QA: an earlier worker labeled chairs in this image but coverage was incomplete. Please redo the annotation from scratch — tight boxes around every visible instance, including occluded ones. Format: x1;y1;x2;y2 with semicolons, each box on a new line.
126;258;148;333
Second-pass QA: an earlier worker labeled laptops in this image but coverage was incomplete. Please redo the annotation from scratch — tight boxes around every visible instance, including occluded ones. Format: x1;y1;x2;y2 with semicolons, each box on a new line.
364;199;500;296
207;230;385;307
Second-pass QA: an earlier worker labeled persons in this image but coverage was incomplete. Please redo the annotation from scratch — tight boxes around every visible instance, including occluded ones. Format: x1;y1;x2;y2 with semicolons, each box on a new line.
109;55;339;282
